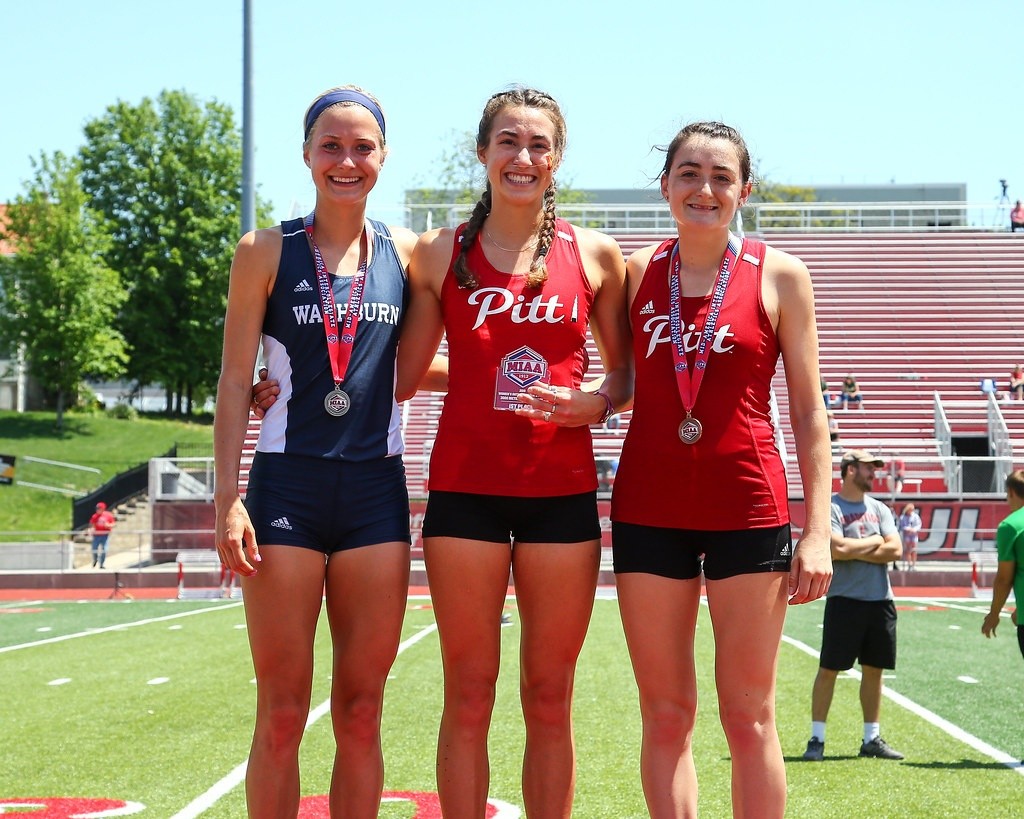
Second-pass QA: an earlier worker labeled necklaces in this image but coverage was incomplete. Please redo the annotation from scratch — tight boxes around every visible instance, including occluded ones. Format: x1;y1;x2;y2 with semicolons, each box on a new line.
483;225;542;252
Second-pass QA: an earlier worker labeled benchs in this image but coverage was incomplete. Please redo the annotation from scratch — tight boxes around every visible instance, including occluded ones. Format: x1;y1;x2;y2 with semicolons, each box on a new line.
599;548;615;572
896;480;922;491
969;552;998;571
176;549;221;570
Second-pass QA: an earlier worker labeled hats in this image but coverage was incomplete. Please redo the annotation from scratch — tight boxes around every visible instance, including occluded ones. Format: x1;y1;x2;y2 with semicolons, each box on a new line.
96;502;106;509
841;451;884;468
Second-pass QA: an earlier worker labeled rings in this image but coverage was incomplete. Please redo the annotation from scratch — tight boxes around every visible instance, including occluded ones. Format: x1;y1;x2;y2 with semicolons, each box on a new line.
254;395;259;404
542;391;558;421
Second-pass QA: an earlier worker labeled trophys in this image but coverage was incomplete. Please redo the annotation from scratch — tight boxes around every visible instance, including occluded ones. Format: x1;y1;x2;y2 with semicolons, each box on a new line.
494;367;551;411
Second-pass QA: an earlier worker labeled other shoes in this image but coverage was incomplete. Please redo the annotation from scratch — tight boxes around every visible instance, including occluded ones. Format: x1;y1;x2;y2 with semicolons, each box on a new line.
93;560;106;569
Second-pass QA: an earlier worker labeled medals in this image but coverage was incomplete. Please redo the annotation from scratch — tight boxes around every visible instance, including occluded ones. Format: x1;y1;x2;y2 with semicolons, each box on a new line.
324;382;350;417
678;409;702;444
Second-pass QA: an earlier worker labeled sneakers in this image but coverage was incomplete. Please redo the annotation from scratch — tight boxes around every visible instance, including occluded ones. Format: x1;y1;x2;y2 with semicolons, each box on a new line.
859;735;905;760
803;736;825;760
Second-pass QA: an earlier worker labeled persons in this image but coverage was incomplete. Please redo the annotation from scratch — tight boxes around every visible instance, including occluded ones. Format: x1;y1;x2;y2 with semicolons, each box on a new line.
982;468;1024;657
582;122;833;819
887;502;922;571
1011;200;1024;233
213;85;449;819
251;83;635;819
1010;366;1024;400
819;373;863;440
803;449;903;760
882;449;906;492
87;502;116;569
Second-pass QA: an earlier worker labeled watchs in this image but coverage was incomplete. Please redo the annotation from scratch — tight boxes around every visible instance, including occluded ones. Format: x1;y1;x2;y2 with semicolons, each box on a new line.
590;391;615;423
